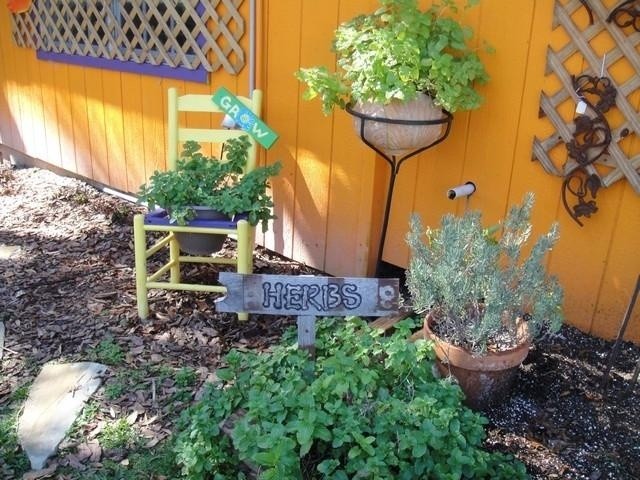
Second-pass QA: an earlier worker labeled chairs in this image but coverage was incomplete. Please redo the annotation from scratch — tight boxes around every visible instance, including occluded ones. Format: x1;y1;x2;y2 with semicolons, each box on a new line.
133;88;263;320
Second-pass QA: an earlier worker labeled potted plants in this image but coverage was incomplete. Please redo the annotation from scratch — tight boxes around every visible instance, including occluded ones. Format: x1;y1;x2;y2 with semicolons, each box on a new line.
404;193;564;412
296;0;497;156
132;135;284;257
